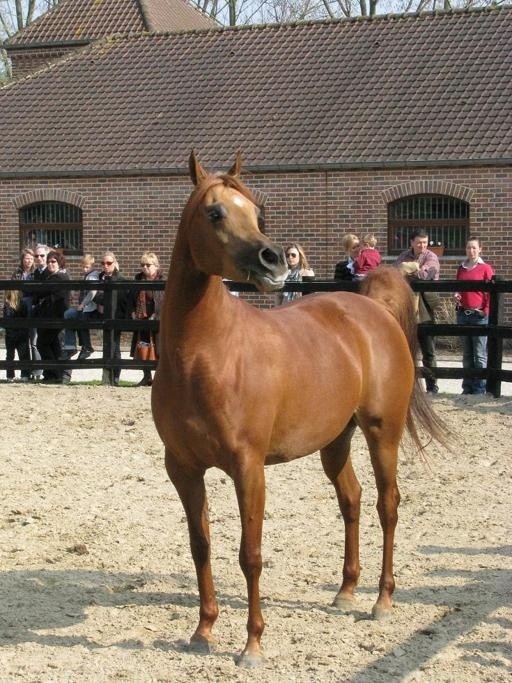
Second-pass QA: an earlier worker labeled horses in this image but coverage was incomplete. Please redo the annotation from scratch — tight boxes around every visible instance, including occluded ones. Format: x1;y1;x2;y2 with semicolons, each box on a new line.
148;148;465;672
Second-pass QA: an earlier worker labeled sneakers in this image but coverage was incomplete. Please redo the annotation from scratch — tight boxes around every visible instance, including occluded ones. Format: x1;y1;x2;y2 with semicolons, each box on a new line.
6;375;70;385
59;349;76;360
78;347;93;359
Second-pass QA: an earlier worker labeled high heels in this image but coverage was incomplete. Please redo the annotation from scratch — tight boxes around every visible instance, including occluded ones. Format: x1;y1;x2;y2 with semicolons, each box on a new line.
136;379;151;387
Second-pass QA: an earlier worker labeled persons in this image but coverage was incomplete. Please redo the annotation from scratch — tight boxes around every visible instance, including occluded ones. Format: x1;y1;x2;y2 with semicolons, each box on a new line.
333;233;361;291
453;236;495;394
275;241;314;306
392;227;439;394
1;243;167;384
353;233;381;279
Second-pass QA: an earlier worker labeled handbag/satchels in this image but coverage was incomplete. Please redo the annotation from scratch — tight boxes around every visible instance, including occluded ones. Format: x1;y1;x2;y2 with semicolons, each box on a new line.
134;342;156;361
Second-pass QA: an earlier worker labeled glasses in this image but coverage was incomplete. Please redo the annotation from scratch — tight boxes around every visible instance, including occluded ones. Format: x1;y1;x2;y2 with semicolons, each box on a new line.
141;264;155;268
286;253;296;259
101;261;113;266
33;254;45;258
